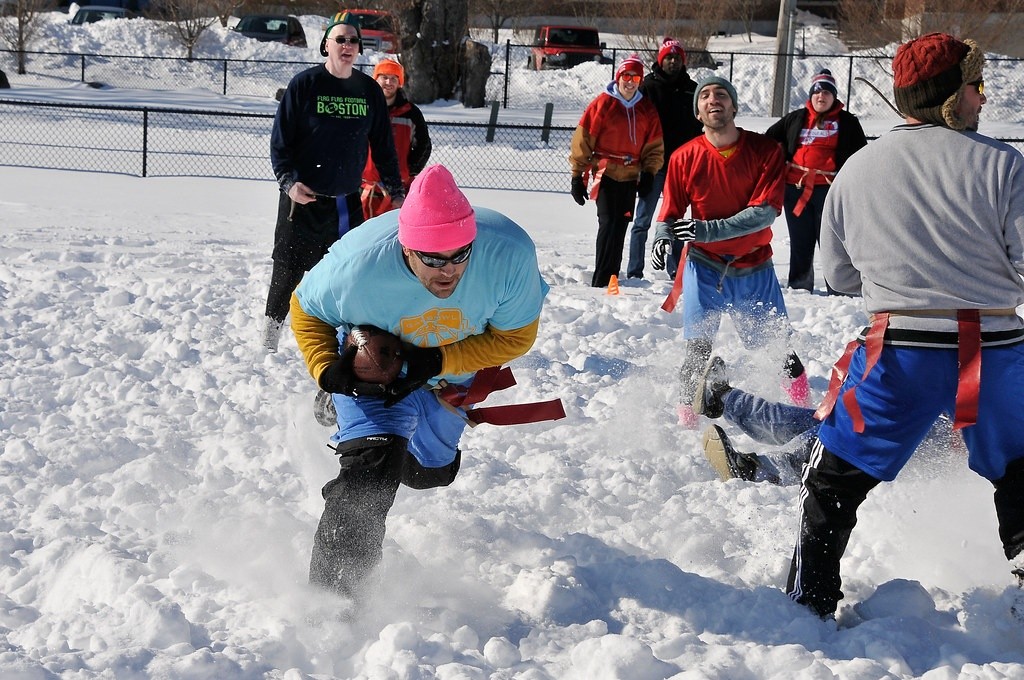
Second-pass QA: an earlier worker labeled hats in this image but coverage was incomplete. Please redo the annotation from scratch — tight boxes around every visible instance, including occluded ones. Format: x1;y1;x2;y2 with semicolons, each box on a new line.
891;33;987;131
693;75;738;123
657;37;687;68
320;12;365;57
809;69;838;101
372;58;404;85
397;164;477;252
615;53;645;87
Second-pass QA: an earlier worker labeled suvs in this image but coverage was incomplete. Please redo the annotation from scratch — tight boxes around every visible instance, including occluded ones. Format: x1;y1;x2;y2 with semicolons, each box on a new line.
525;25;614;74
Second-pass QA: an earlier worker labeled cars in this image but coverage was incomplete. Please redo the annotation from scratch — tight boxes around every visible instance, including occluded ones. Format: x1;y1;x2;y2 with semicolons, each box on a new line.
336;9;396;54
64;6;133;28
232;15;309;48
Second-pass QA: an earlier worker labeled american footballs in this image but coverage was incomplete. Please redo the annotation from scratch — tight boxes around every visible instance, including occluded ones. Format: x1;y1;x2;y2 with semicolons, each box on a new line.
343;325;404;386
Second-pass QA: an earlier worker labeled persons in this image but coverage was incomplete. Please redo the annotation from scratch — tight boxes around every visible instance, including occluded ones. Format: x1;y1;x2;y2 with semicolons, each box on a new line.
653;77;814;428
569;51;666;288
765;68;868;297
688;32;1022;630
262;10;432;356
289;165;568;625
626;36;703;281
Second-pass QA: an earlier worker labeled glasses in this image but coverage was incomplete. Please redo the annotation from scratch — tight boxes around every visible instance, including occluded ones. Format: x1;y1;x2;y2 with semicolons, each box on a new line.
413;241;473;268
966;80;984;96
326;35;361;44
620;73;641;83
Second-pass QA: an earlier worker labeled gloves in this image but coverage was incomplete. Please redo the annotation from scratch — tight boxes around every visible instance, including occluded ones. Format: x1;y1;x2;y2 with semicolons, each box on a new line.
669;219;696;240
570;176;589;206
321;346;442;407
651;239;672;270
638;172;654;197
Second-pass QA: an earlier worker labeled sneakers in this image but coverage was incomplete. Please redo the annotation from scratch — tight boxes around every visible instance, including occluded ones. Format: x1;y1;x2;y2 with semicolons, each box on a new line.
313;389;337;427
695;356;729;418
702;424;757;482
